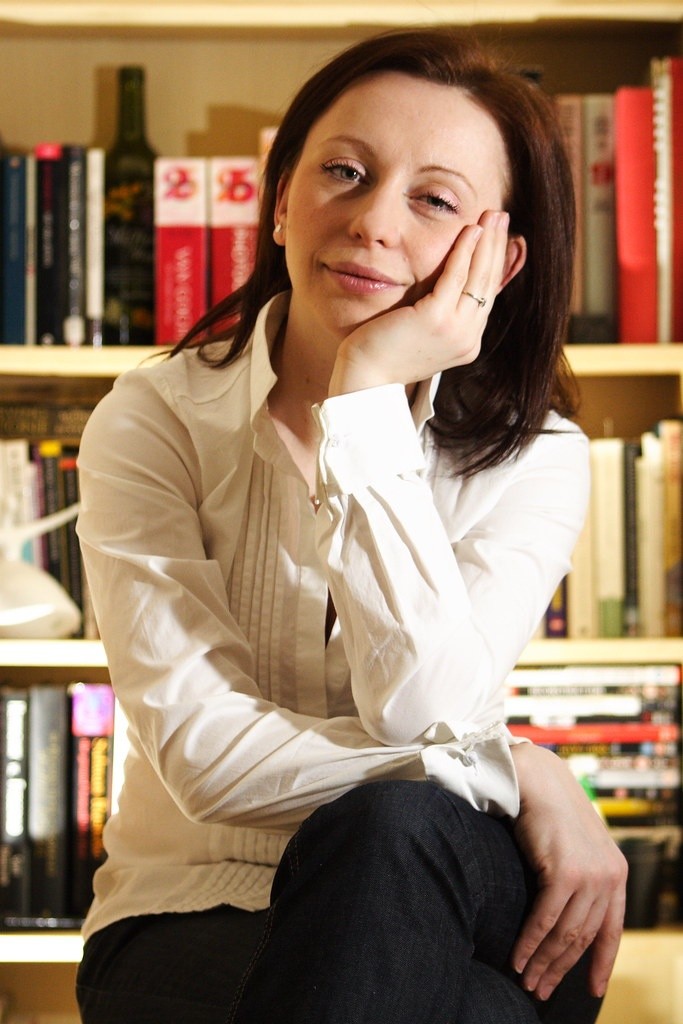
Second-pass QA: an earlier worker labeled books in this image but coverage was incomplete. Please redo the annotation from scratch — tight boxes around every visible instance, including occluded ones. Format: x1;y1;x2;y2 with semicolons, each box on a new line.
505;60;683;923
0;64;277;933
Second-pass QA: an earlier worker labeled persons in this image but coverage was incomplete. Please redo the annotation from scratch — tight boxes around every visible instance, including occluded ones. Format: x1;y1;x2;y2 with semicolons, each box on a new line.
74;28;629;1024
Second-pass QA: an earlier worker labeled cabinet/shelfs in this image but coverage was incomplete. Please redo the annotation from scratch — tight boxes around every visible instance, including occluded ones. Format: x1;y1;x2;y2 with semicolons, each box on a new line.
11;0;682;964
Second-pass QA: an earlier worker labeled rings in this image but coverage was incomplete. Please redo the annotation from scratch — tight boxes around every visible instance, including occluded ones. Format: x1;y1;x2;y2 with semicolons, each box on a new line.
462;291;487;307
